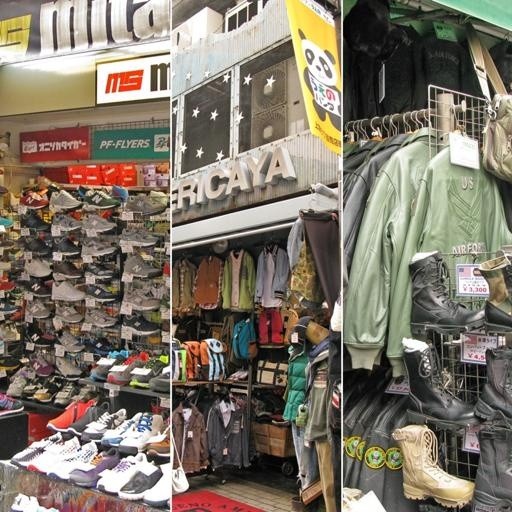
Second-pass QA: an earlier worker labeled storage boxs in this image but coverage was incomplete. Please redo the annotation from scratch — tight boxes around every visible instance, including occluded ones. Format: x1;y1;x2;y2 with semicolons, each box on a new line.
251;419;295;457
40;164;169;187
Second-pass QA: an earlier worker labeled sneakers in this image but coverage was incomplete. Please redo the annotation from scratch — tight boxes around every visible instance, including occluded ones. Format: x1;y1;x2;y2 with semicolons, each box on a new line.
0;183;170;416
10;399;170;507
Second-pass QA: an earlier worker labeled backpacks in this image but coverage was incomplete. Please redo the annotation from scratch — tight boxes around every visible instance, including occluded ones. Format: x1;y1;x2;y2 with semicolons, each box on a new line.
171;305;226;383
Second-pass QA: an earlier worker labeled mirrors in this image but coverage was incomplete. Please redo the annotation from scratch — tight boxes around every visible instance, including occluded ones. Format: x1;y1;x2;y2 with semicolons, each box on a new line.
169;1;342;511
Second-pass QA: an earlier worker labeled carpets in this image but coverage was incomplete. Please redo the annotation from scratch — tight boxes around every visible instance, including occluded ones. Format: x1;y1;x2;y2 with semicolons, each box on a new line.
173;485;265;512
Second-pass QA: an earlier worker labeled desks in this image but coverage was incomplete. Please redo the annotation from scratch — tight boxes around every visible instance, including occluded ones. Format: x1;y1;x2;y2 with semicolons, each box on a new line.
1;462;169;512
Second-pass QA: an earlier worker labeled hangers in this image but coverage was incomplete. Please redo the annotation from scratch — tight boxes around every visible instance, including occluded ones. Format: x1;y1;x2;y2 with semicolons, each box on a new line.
177;233;278;263
343;375;410;439
345;104;468;143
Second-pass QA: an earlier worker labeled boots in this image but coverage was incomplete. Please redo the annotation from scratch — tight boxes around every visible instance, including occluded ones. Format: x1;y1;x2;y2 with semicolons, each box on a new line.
475;425;512;512
409;251;485;336
472;345;512;429
392;424;475;509
475;248;512;332
403;343;486;431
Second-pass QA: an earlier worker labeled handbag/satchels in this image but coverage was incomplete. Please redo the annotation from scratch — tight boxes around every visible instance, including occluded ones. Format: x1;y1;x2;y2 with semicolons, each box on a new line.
256;360;288;387
232;319;258;360
258;308;299;345
480;91;511;183
295;403;309;427
172;467;189;496
